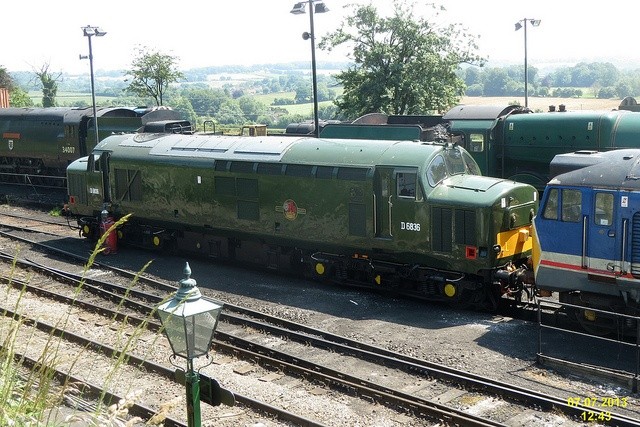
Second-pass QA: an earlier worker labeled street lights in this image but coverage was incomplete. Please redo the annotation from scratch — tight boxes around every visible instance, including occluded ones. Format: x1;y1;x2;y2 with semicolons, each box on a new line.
78;23;108;152
291;0;329;138
0;105;640;217
153;260;226;427
515;17;541;107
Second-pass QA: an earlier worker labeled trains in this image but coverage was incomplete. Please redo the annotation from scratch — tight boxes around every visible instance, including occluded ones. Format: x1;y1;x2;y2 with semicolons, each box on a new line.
64;122;640;347
